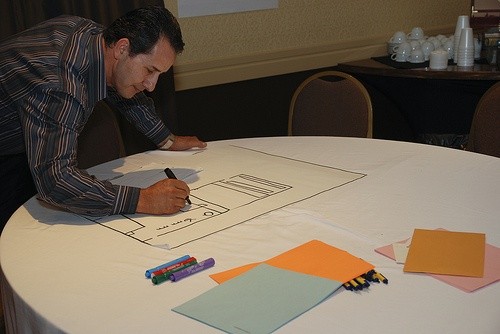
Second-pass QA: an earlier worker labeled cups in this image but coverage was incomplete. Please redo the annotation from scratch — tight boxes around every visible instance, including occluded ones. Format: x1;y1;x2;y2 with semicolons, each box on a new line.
387;16;481;69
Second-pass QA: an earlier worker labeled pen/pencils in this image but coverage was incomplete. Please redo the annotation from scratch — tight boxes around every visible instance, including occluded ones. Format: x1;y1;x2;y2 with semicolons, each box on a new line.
343;269;388;291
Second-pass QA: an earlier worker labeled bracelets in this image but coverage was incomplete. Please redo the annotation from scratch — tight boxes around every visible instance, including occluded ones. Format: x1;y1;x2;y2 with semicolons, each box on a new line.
156;133;176;150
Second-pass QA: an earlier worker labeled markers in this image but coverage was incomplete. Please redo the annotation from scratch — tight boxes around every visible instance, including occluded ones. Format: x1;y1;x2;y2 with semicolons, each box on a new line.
164;168;191;204
145;255;197;285
170;257;215;282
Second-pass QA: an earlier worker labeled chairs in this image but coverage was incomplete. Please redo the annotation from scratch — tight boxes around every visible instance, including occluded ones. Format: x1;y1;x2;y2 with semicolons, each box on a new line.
469;81;500;158
76;100;127;170
288;71;373;138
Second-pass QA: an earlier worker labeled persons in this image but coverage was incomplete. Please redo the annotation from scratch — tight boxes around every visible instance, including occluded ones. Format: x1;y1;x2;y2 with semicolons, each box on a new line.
0;6;207;215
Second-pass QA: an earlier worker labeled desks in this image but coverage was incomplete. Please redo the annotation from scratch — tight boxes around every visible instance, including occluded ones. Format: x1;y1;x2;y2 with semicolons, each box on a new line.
337;54;500;80
0;136;500;334
